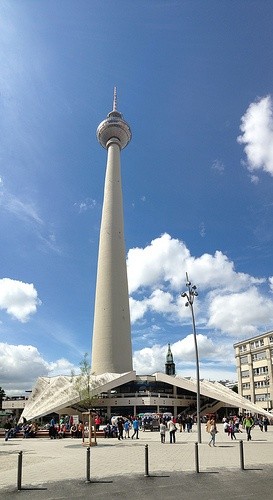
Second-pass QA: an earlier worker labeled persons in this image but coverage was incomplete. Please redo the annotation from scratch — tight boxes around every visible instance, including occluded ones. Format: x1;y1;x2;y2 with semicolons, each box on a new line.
44;415;84;439
94;416;100;431
207;414;216;447
179;416;194;432
160;416;177;443
139;415;154;431
259;416;269;432
117;415;139;440
223;414;254;441
4;417;38;441
104;424;118;437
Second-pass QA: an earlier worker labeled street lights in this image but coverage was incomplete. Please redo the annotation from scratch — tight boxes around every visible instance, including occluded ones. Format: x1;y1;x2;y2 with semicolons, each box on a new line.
180;269;202;443
263;375;270;413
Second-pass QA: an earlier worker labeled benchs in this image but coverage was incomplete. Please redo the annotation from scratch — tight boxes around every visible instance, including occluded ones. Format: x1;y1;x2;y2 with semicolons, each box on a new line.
0;429;105;438
